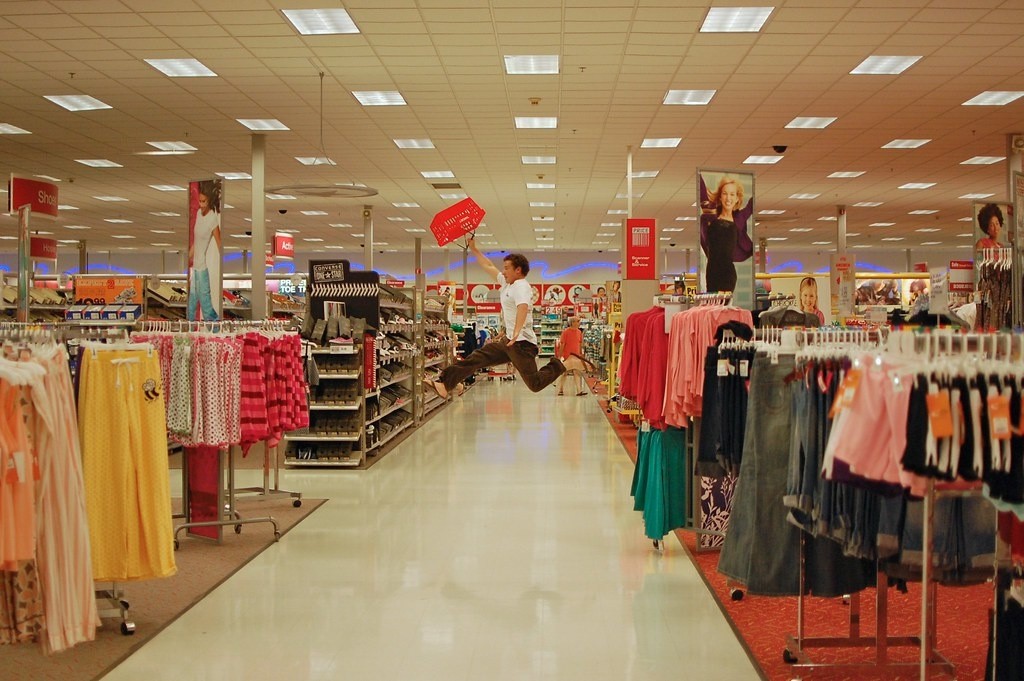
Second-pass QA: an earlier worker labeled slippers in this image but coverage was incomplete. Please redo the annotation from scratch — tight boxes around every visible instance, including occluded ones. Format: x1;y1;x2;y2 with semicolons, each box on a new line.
422;379;447;401
570;352;595;374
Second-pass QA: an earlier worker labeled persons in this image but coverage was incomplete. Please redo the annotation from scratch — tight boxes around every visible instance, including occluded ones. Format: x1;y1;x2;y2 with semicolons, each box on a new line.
554;316;588;396
597;287;606;299
975;206;1008;251
187;179;221;333
800;277;827;326
910;280;926;301
675;281;686;297
611;281;622;303
419;239;597;399
699;172;755;304
468;323;492;342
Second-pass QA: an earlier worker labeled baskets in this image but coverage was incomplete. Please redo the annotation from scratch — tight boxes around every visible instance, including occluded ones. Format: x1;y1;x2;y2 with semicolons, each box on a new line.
430;197;486;248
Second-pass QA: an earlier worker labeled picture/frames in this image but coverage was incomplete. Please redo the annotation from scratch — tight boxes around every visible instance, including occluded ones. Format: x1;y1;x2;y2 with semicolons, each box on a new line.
972;200;1017;292
185;176;225;322
695;165;756;312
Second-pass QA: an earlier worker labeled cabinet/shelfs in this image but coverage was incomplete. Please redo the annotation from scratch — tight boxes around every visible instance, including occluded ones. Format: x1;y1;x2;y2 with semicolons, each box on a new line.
0;303;73;331
449;304;643;415
282;286;455;468
143;280;306;332
64;312;144;386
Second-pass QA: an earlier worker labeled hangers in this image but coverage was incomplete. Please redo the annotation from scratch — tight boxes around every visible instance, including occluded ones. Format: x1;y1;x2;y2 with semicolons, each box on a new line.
947;292;1012;313
653;290;1024;480
0;317;317;358
977;248;1013;272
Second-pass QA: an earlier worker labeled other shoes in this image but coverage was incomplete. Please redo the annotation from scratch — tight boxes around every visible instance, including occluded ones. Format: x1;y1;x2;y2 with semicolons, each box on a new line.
576;391;588;396
558;391;563;395
